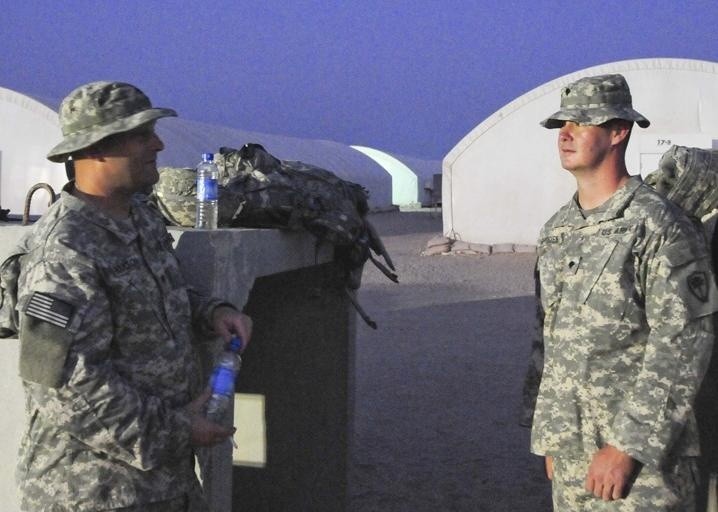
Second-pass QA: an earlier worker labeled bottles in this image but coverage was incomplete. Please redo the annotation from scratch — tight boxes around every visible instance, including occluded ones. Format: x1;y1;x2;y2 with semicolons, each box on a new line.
194;152;219;230
200;333;243;423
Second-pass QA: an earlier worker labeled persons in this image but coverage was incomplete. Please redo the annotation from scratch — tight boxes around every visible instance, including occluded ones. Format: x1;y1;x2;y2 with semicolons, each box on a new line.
17;81;254;512
520;74;717;511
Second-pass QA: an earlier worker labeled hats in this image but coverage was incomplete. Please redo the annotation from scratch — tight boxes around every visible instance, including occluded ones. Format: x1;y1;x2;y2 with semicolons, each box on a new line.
539;71;652;130
46;80;179;163
